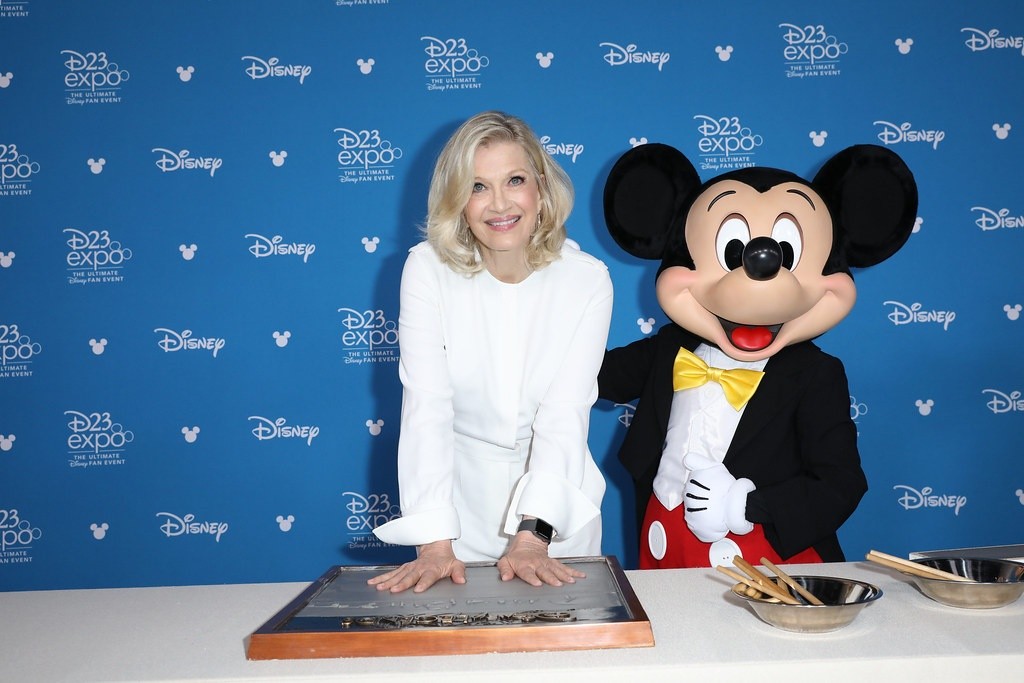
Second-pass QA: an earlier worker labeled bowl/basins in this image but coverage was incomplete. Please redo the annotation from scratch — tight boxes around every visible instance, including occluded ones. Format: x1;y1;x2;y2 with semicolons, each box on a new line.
732;575;883;633
895;557;1024;609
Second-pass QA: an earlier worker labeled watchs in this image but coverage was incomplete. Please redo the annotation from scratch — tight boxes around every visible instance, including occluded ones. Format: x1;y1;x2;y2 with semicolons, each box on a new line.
518;517;553;546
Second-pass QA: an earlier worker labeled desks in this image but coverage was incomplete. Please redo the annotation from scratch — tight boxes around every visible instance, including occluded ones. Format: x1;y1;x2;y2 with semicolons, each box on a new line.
0;556;1024;683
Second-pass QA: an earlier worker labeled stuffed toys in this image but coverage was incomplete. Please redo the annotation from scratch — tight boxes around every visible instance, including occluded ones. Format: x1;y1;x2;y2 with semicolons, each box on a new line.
598;144;920;568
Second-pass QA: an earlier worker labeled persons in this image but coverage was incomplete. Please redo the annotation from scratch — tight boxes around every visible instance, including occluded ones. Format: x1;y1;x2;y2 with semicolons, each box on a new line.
366;110;614;593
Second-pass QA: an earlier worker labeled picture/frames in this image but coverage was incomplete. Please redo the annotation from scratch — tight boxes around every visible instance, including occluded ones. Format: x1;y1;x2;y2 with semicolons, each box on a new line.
247;555;655;660
